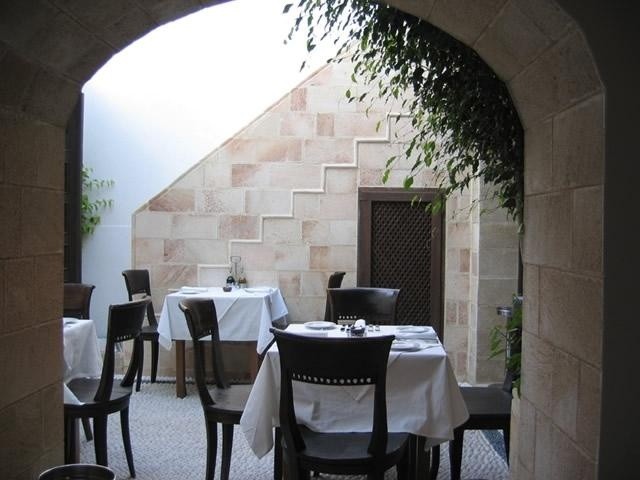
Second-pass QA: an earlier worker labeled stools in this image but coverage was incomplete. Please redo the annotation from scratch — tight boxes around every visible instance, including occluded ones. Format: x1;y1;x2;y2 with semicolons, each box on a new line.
452;384;510;478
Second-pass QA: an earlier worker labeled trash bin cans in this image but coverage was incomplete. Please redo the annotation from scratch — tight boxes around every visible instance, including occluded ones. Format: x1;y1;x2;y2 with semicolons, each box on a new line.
38;463;116;479
498;308;522;385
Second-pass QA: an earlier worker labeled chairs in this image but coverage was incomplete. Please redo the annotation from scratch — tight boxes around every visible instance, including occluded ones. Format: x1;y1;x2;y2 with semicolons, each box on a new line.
323;271;346;323
178;294;273;480
38;268;157;480
325;286;401;328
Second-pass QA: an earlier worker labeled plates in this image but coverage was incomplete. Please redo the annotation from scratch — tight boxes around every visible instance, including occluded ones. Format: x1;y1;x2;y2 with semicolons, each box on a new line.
382;339;422;353
394;324;430;333
304;320;336;330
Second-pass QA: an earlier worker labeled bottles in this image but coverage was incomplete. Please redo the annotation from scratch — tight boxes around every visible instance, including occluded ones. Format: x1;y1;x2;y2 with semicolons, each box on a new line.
225;270;248;290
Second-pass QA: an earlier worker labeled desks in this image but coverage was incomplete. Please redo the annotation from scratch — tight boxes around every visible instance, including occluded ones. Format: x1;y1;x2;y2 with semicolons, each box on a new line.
238;320;469;479
